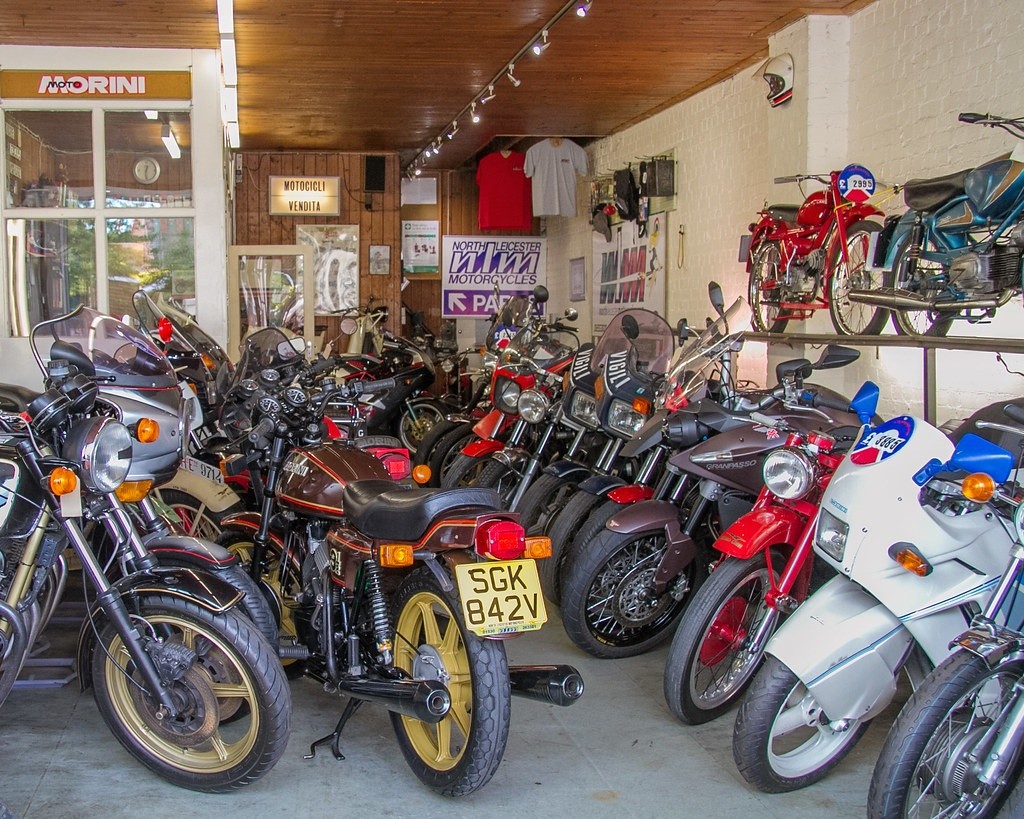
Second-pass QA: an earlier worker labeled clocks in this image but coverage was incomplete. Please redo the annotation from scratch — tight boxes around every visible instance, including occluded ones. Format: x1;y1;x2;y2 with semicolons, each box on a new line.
132;156;161;184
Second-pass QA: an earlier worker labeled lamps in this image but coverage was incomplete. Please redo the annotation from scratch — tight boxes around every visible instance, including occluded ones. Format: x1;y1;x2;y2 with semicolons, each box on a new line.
144;111;165;124
426;142;436;157
434;137;443;154
407;167;416;179
507;64;520;87
415;158;421;176
533;31;551;56
447;121;459;139
481;86;495;104
407;171;412;181
161;124;182;159
577;0;594;17
470;103;479;123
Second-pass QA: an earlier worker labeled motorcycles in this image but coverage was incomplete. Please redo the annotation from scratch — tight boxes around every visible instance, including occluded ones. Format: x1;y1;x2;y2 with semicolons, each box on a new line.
731;381;1024;819
745;170;893;335
0;282;867;799
848;112;1024;337
